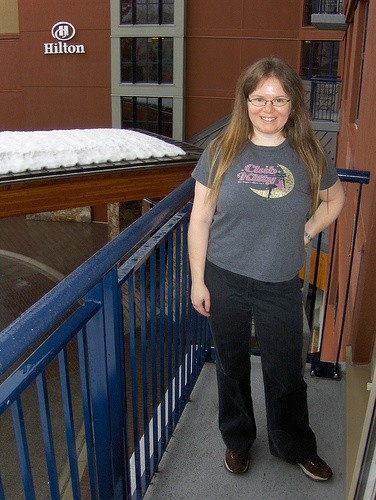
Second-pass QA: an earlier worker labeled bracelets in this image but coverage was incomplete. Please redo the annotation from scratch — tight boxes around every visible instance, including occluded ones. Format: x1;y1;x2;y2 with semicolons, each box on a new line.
304;232;314;243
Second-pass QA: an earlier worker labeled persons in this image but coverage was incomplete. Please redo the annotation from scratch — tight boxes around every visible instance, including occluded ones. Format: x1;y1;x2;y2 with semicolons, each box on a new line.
187;57;346;482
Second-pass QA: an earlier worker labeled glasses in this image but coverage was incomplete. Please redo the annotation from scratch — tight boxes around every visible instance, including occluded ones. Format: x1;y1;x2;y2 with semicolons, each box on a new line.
247;96;291;107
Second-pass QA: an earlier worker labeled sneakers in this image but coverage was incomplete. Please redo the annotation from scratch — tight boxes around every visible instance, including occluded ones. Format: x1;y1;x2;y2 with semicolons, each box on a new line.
297;455;333;480
225;448;250;473
